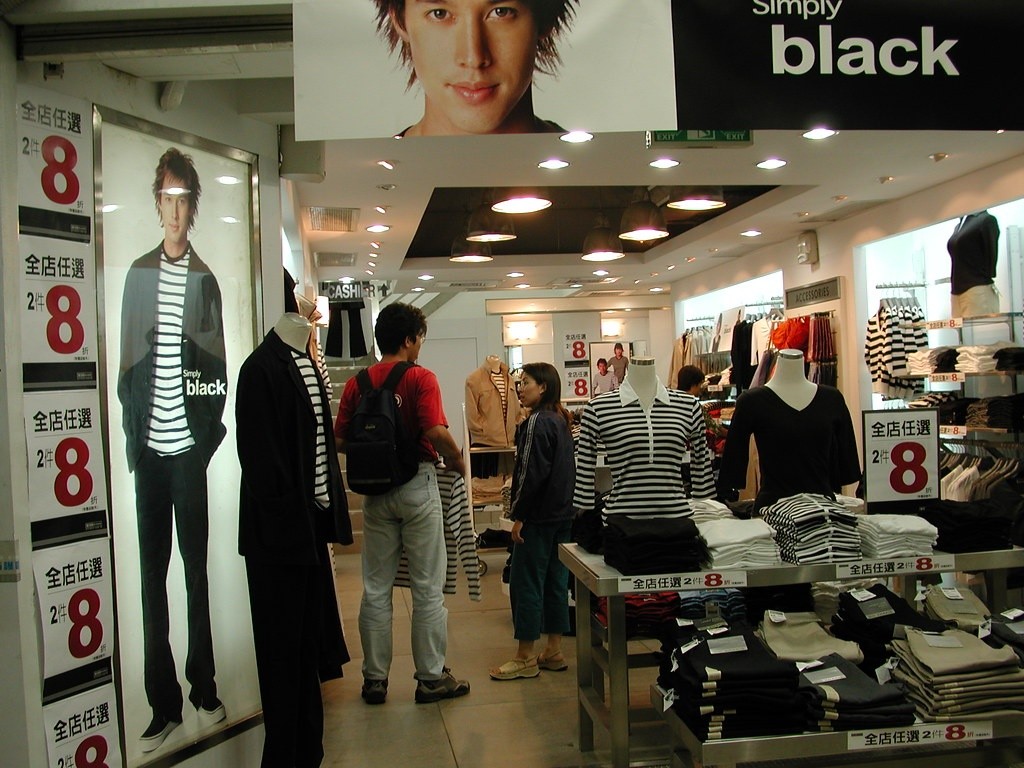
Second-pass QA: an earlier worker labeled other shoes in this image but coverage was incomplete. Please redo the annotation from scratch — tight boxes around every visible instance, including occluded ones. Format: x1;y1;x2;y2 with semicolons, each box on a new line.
196;697;225;722
138;714;183;753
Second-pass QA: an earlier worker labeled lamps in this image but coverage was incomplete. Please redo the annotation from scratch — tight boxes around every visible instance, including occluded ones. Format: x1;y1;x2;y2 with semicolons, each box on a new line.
366;128;1004;293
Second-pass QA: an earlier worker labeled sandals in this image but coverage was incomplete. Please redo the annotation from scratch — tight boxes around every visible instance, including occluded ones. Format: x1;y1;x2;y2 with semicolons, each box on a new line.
490;655;541;680
537;650;568;671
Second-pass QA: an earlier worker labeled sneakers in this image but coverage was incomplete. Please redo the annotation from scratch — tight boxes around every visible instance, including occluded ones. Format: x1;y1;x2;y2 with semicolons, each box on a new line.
415;666;471;704
361;677;389;705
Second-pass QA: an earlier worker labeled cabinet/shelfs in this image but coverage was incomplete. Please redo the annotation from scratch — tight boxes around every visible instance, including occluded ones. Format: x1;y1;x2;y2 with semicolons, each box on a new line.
897;312;1024;434
468;445;517;552
558;542;1024;768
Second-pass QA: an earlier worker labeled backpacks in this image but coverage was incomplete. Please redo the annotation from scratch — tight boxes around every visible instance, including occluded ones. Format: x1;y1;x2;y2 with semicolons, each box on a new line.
346;360;420;496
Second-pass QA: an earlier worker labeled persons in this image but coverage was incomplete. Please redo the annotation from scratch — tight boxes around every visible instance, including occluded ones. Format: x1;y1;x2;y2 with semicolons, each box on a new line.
606;343;628;384
677;365;705;499
235;311;354;768
332;302;470;705
572;355;717;527
718;349;861;519
947;210;1000;319
591;358;618;399
464;354;521;448
372;0;581;137
489;362;576;680
116;147;228;753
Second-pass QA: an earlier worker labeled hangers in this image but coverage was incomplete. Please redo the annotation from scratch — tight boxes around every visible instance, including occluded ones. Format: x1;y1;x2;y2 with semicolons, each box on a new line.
683;296;784;340
939;438;1024;480
873;281;922;314
